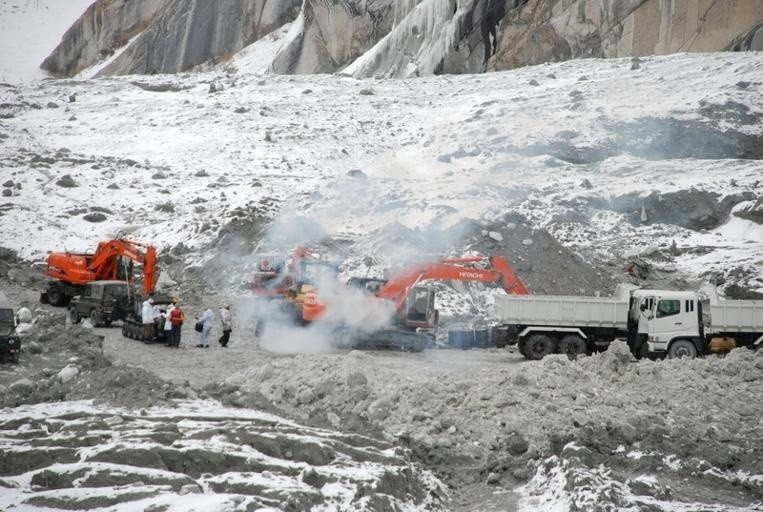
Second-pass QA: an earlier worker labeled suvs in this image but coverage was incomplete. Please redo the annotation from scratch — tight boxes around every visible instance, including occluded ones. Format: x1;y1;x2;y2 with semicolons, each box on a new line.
0;306;24;365
65;279;138;329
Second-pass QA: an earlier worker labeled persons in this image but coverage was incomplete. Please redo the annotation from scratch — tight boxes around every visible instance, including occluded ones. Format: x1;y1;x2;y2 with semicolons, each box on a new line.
168;300;185;349
194;306;215;349
632;304;651;358
141;296;159;344
217;303;233;347
162;299;173;347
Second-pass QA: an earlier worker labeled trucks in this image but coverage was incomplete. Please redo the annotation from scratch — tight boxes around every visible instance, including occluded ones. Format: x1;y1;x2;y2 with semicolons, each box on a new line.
628;284;763;363
492;280;645;361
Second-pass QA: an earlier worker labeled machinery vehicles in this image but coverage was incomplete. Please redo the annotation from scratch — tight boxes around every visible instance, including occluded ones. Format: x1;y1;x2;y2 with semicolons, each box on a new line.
120;292;179;343
39;236;162;309
324;250;532;356
247;236;361;348
